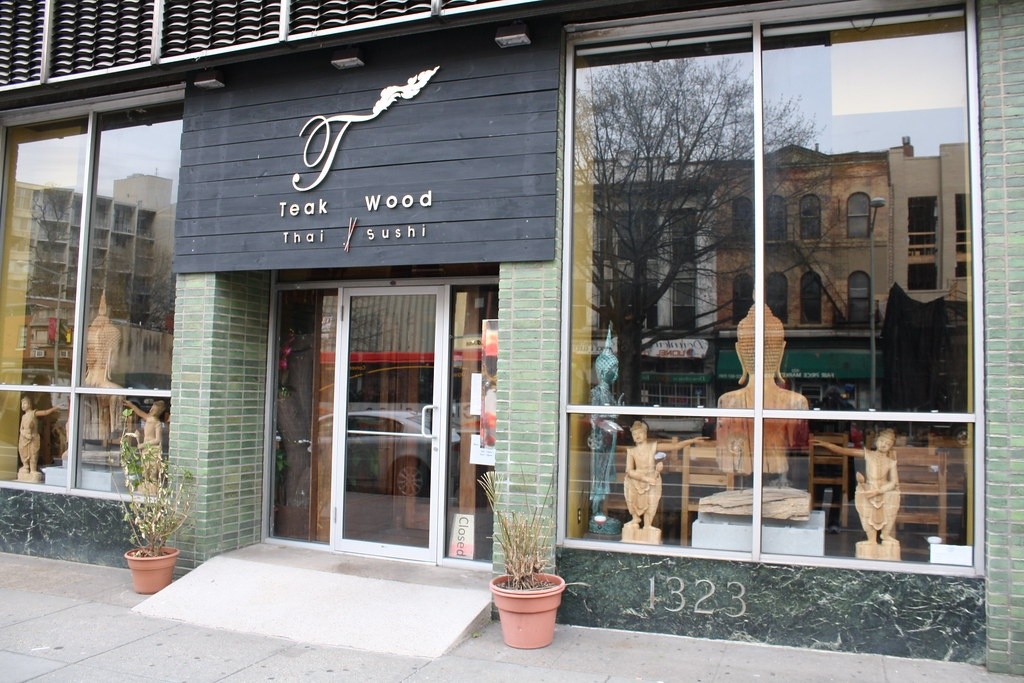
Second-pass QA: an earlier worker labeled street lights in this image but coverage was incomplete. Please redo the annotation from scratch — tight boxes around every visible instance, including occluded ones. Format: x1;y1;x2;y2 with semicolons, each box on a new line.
867;196;887;412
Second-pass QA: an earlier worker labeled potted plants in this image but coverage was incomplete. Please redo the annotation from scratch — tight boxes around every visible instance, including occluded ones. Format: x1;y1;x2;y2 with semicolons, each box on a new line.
476;467;566;648
109;440;200;594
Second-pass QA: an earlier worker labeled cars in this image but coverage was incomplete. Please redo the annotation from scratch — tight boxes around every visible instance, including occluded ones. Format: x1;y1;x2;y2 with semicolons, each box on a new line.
0;368;169;451
913;411;974;448
702;402;864;458
579;414;627;452
318;409;461;498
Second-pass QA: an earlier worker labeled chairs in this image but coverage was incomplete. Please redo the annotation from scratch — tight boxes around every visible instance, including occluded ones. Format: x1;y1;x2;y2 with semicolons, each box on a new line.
603;431;967;557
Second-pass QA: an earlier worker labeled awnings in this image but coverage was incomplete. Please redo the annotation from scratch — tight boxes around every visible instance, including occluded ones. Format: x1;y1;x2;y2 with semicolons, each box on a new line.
717;349;884;380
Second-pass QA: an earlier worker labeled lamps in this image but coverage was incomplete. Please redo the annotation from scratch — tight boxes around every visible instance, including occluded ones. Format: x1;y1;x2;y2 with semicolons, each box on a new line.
496;32;531;49
331;51;367;71
194;74;226;89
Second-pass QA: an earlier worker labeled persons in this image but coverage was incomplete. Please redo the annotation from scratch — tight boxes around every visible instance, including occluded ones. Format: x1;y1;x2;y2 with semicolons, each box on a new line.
590;327;619;522
808;428;901;546
624;421;709;530
18;396;65;475
123;399;165;468
82;289;125;440
717;303;808;475
813;386;857;534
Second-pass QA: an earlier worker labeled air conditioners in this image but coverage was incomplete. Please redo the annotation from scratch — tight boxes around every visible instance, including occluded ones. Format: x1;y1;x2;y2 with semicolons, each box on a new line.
60;350;69;359
35;350;46;358
